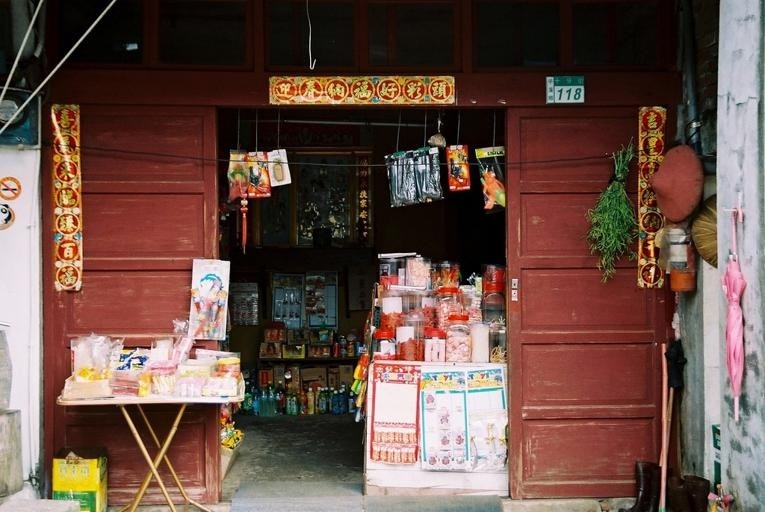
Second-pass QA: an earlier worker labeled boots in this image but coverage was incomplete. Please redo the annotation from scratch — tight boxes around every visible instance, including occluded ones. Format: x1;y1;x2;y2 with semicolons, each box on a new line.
618;462;660;512
682;476;709;511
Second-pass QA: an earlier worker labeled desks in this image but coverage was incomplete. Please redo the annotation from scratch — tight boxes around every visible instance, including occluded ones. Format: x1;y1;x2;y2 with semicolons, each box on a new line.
56;382;246;512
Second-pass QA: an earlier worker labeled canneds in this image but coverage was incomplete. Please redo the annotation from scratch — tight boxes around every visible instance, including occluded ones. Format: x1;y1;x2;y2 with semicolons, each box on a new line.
347;342;355;357
340;347;347;358
355;342;361;356
339;336;346;347
332;343;340;357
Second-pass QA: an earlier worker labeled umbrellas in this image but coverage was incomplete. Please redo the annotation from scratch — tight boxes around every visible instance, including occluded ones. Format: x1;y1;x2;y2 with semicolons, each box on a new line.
722;205;748;423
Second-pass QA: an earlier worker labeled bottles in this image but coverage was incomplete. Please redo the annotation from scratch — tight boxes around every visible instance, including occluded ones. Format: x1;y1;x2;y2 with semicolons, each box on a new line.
241;372;357;416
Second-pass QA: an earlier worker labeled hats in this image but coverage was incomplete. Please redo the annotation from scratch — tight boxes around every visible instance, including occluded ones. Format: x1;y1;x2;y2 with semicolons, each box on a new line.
649;146;703;223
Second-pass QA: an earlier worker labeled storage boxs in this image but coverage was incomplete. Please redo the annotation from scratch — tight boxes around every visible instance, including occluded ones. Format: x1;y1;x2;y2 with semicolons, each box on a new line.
299;367;326;390
52;457;108;512
